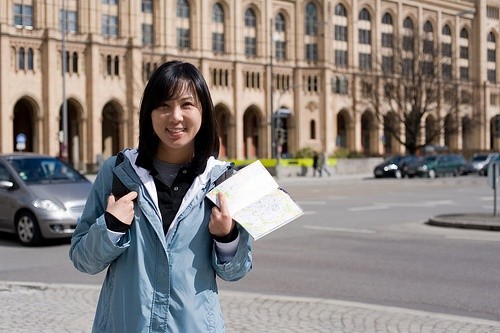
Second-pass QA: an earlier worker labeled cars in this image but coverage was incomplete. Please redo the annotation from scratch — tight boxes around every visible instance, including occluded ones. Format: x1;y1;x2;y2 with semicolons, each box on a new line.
374;152;500;179
0;152;93;246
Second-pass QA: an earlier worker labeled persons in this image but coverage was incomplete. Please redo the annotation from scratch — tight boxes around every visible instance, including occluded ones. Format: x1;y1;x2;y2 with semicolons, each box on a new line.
69;59;254;333
311;150;332;178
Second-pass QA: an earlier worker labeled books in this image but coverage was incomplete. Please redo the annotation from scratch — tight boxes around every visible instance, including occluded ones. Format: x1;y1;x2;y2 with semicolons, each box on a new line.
206;160;304;241
